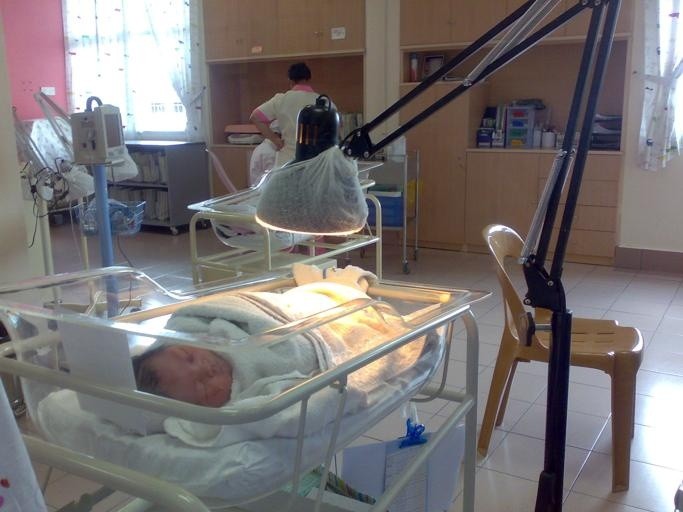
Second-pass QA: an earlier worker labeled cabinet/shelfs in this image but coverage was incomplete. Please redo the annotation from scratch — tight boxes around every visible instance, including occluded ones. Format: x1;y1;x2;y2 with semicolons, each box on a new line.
359;143;421;273
85;139;209;235
386;0;637;269
201;1;386;250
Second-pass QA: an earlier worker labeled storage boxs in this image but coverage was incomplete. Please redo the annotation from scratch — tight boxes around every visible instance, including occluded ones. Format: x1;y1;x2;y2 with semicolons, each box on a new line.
369;183;403;227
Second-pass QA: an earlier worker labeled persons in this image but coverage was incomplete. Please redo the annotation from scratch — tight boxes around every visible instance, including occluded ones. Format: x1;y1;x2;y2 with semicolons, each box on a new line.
131;340;232;408
249;62;343;168
249;119;281;188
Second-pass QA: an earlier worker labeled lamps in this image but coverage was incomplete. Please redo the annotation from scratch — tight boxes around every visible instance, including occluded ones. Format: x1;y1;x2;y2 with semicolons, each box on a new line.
252;0;622;512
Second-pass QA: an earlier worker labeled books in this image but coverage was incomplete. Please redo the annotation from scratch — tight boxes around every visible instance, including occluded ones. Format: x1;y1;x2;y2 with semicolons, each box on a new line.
589;112;622;151
337;108;362;141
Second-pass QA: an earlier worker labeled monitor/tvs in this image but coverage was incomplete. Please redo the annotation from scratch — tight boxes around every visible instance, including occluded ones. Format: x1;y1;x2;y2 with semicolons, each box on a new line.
70;104;125;165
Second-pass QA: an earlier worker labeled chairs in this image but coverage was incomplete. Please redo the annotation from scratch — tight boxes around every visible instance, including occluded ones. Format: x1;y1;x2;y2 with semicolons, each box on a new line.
477;223;643;494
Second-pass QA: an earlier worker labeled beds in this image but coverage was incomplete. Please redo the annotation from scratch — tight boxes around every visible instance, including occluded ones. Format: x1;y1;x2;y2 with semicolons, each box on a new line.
0;265;497;511
186;158;382;302
